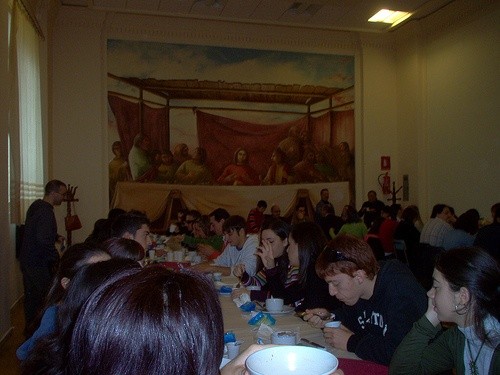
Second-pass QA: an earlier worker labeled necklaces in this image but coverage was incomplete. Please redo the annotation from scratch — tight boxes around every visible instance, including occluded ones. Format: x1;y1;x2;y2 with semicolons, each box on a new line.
467;329;491;375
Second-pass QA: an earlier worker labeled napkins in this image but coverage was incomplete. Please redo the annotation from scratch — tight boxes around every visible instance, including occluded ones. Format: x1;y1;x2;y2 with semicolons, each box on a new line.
337;357;388;375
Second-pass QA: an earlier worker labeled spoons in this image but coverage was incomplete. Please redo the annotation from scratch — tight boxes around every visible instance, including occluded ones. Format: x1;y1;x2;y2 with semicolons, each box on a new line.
294;311;335;321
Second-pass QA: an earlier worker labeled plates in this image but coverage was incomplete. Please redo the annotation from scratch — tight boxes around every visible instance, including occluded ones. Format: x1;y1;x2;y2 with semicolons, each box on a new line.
262;306;294;315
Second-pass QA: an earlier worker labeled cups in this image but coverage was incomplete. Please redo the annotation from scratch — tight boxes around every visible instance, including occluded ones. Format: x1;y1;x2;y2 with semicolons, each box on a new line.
167;251;183;261
232;288;241;302
213;272;222;281
227;342;240;359
324;321;341;328
170;225;176;232
149;250;155;259
185;252;201;262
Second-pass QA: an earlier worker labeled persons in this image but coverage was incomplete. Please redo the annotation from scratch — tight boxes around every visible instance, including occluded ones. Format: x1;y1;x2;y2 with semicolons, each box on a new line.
15;208;153;375
302;233;428;365
66;265;280;375
166;188;500;307
389;244;500;375
19;179;66;340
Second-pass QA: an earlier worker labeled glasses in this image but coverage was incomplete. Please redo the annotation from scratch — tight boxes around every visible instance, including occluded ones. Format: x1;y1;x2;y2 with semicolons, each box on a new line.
184;220;194;224
323;244;350;263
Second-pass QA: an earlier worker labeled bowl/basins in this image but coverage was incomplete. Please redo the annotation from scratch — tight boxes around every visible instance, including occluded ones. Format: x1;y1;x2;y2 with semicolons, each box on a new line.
265;298;283;312
246;345;339;375
247;286;260;290
272;330;296;345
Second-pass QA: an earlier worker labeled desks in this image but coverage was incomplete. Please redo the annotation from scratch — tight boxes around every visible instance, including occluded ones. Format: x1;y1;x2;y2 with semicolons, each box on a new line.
191;260;391;375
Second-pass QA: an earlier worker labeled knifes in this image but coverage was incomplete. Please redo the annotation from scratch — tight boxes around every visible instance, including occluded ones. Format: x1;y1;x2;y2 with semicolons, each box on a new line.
301;338;325;348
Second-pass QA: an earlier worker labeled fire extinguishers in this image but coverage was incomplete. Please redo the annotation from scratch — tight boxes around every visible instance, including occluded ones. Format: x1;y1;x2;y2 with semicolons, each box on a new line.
378;168;390;194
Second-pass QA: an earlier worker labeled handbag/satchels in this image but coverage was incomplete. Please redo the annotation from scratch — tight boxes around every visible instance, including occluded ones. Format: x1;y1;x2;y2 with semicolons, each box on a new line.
65;202;81;231
